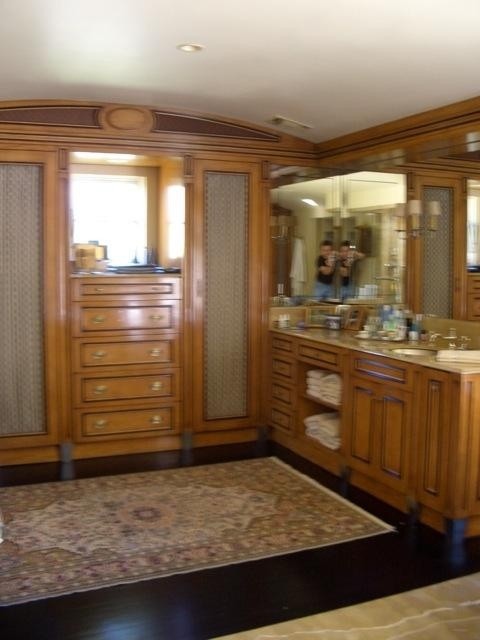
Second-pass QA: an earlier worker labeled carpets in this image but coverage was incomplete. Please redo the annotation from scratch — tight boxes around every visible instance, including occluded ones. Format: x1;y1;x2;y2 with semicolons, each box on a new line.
0;455;397;606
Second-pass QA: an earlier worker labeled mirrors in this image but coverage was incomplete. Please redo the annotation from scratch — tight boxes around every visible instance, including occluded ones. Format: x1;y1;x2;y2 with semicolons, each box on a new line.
271;131;480;322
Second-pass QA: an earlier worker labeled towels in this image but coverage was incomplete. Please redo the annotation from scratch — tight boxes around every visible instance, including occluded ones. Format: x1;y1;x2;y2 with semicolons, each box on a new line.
301;367;342;450
436;350;480;364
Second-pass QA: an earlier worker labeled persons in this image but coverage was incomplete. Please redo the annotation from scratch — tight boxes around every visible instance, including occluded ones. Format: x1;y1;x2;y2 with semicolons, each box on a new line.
335;239;368;298
311;238;337;300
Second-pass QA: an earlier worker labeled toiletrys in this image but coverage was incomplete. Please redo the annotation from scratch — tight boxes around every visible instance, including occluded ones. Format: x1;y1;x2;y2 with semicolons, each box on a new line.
356;304;430;342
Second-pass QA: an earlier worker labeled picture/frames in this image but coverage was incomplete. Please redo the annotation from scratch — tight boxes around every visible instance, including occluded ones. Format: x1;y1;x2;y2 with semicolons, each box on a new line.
343;307;367;332
305;304;336;328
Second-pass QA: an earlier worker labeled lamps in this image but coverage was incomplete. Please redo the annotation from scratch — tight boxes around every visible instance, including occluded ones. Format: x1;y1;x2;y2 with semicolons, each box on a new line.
394;201;441;240
271;216;298;240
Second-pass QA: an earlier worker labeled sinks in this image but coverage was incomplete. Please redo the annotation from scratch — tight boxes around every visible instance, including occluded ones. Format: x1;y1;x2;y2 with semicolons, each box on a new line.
103;264;181;274
389;347;437;356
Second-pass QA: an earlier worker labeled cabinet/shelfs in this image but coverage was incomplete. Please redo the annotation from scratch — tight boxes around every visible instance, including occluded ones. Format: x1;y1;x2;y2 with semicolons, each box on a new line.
269;331;480;542
69;275;186;442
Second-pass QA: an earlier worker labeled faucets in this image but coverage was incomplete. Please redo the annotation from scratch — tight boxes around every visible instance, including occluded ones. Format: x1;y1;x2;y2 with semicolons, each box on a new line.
131;247;139;262
428;328;458;350
